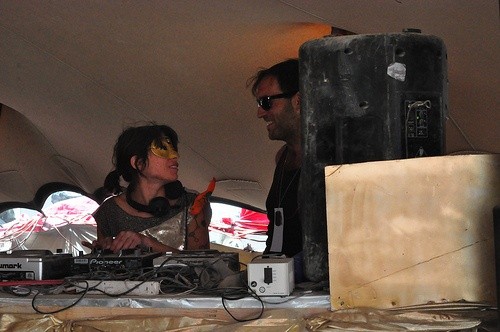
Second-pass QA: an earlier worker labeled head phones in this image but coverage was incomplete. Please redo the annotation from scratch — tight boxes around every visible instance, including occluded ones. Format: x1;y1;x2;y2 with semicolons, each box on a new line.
126;179;183;218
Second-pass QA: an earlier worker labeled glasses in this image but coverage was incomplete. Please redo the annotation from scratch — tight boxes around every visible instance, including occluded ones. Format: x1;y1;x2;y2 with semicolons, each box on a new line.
256;93;296;111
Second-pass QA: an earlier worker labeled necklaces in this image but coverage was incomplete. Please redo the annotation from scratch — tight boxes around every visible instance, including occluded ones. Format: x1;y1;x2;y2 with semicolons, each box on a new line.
275;147;301;227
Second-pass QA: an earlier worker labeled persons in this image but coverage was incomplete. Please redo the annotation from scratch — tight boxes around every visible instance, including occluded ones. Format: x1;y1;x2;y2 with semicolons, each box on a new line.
82;123;213;254
246;58;306;257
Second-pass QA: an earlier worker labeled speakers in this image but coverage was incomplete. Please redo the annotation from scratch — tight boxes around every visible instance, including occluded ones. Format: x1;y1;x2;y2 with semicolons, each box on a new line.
298;30;447;287
